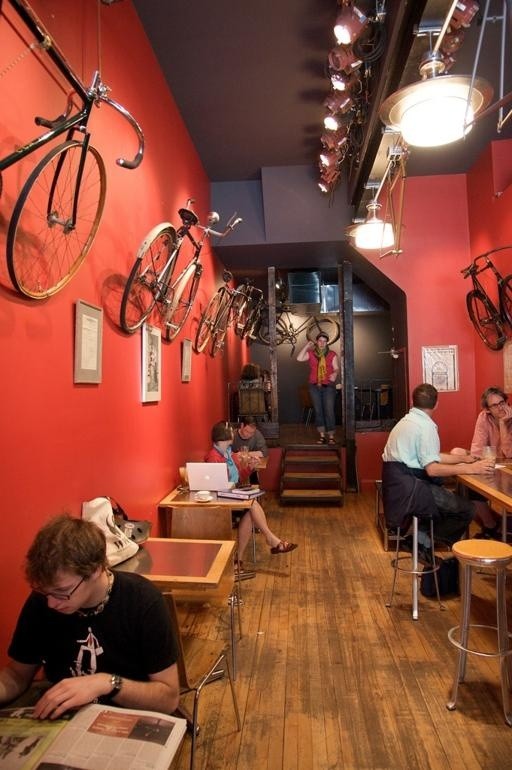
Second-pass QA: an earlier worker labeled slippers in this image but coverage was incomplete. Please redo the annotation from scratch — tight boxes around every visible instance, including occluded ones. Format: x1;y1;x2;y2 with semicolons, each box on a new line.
234;559;244;574
271;540;298;554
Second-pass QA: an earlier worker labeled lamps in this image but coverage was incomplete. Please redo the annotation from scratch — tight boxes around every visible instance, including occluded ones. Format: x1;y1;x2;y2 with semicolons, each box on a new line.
313;8;370;194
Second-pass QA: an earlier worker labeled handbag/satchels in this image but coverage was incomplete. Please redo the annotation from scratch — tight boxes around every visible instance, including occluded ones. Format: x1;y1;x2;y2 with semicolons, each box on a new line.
420;557;459;597
81;496;140;568
109;497;150;544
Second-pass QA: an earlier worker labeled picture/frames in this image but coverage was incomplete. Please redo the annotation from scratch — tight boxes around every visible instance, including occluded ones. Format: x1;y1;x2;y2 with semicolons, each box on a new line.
74;298;105;385
141;322;163;404
180;336;194;384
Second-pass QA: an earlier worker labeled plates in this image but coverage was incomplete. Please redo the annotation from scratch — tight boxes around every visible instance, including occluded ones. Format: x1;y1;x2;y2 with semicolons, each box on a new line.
194;496;212;502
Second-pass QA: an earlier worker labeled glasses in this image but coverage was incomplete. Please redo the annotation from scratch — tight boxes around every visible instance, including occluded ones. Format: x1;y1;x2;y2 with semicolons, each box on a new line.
30;576;86;601
488;399;505;410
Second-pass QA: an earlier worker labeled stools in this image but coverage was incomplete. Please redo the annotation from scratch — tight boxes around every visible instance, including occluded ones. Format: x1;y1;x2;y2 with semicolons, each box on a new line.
385;508;512;729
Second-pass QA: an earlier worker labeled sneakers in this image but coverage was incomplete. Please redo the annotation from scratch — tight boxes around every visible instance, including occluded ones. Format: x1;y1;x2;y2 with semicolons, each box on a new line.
401;534;443;567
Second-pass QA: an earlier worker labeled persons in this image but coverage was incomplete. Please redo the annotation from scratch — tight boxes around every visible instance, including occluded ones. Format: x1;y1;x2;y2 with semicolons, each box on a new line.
382;383;498;564
228;413;269;487
295;332;341;446
205;421;298;577
0;517;182;721
465;386;512;540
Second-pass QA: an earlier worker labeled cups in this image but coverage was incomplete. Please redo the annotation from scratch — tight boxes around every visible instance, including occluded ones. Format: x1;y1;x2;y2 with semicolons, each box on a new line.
195;491;210;499
482;445;496;475
240;446;249;459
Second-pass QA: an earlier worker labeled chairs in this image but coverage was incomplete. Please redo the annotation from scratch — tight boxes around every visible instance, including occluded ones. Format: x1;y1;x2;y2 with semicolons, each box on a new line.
298;385;315;430
162;589;241;770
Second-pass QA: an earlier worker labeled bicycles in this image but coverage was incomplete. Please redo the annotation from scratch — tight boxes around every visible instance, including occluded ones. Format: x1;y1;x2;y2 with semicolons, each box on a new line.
198;265;266;353
0;57;144;324
453;245;512;352
121;186;245;350
261;297;357;358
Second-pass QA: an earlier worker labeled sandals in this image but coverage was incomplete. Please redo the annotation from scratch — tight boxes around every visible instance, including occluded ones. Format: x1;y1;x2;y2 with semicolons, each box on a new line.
328;439;336;444
316;437;327;444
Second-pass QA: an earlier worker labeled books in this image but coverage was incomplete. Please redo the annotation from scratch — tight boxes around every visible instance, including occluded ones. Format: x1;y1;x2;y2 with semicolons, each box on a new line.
218;491;267;502
0;701;189;770
230;485;259;496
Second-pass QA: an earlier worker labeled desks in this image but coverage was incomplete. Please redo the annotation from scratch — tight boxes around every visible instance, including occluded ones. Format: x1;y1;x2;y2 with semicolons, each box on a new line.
115;477;257;696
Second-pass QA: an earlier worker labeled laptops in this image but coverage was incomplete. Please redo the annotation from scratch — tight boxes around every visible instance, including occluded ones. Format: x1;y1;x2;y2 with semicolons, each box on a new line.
185;462;234;492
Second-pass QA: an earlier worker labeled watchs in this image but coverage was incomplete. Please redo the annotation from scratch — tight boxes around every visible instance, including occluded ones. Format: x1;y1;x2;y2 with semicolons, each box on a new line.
98;673;122;704
